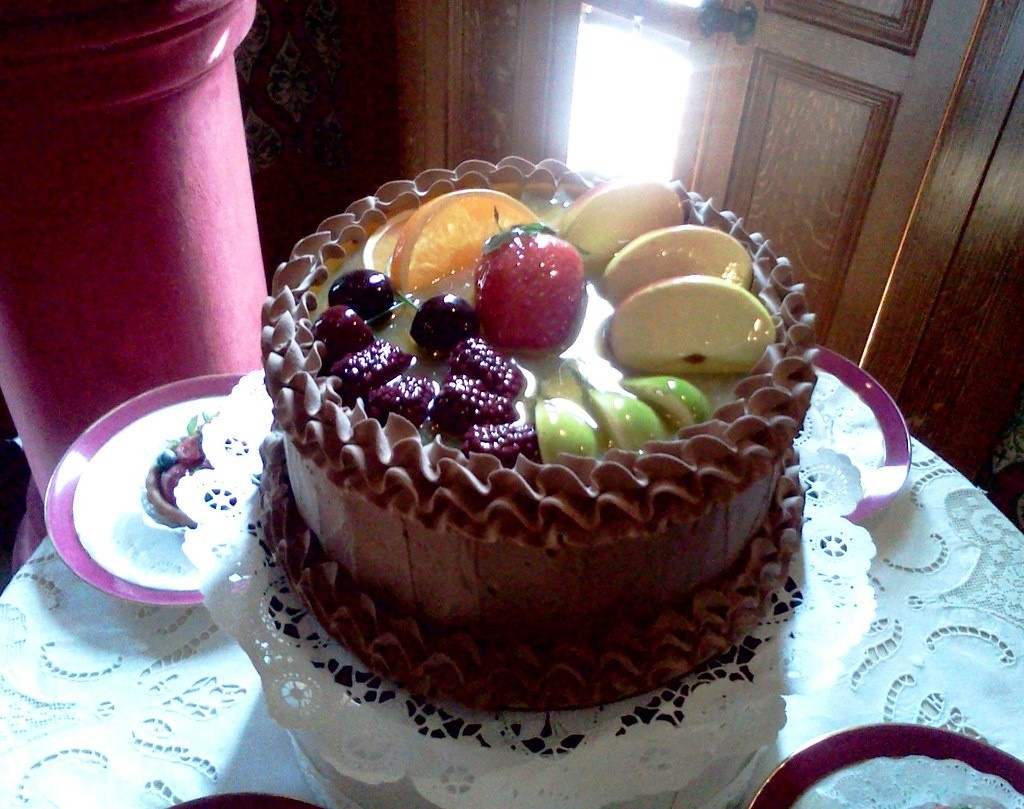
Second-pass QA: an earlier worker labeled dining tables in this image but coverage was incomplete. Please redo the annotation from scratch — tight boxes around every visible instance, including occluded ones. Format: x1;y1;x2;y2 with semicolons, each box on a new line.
0;431;1024;809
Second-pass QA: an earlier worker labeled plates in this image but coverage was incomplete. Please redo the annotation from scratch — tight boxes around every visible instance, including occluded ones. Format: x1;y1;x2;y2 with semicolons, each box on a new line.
750;723;1024;809
46;373;253;606
808;346;912;520
162;791;332;809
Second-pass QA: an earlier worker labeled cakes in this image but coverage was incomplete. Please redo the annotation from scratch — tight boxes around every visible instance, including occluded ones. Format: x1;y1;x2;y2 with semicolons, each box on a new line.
256;155;820;711
